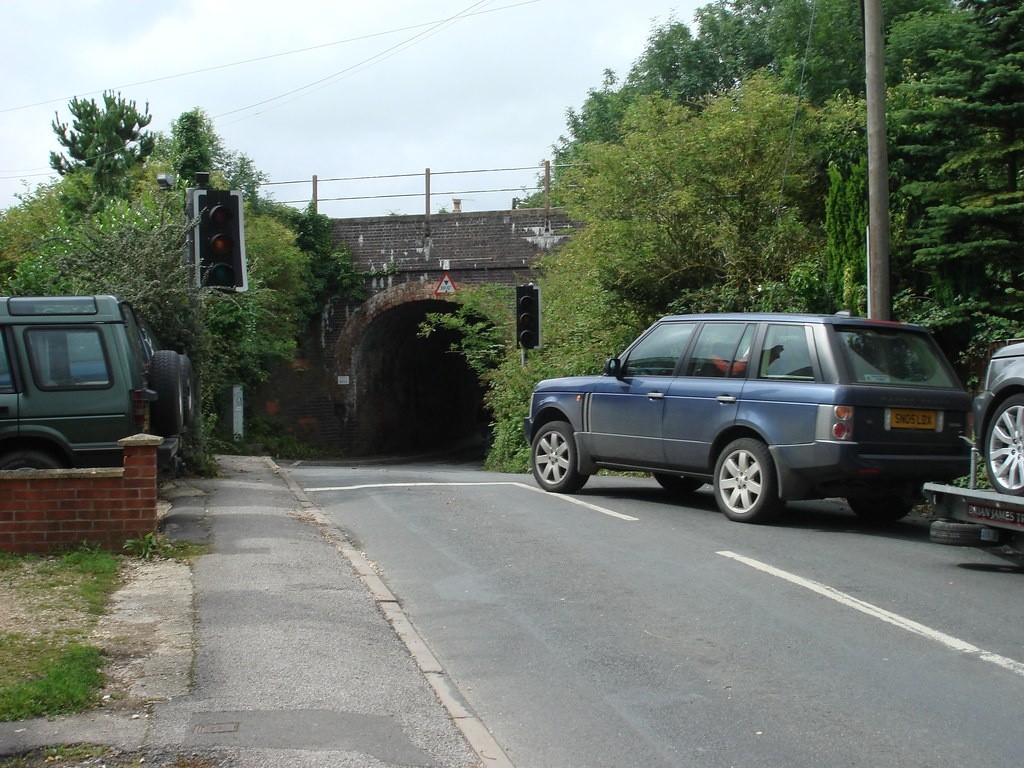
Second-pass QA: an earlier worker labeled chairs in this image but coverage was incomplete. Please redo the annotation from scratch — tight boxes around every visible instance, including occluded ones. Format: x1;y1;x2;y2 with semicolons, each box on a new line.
701;342;747;377
850;345;886;382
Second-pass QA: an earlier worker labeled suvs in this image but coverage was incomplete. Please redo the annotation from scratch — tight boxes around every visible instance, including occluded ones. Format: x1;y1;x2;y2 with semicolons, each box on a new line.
524;312;976;527
0;295;196;485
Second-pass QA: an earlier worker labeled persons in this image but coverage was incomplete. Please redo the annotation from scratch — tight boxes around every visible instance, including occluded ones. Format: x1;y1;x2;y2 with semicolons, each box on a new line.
708;344;747;377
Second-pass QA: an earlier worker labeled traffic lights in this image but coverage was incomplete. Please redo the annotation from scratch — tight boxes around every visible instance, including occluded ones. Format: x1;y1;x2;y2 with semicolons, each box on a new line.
193;188;248;292
516;284;541;349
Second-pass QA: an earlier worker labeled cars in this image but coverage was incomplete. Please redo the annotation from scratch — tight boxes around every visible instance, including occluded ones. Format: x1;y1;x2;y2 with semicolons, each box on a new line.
971;337;1024;496
